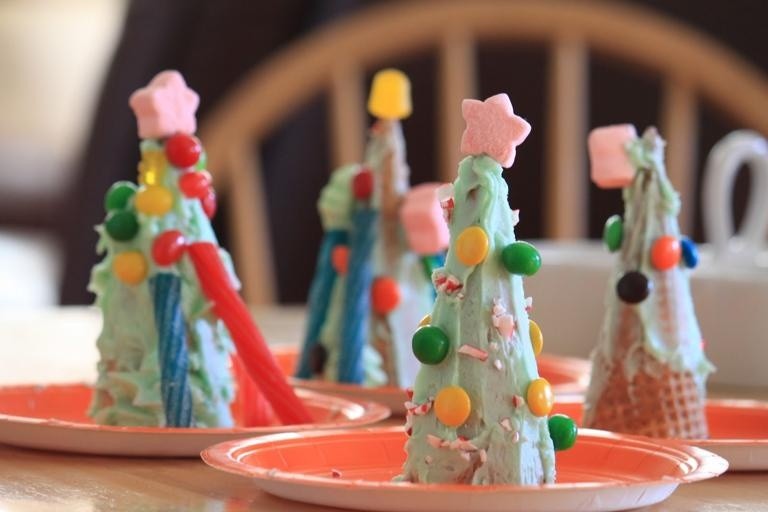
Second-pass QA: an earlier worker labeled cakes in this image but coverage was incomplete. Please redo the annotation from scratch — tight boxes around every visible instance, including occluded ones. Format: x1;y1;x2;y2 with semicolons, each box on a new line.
399;93;577;485
582;119;716;436
86;69;315;423
298;162;357;380
336;68;449;386
